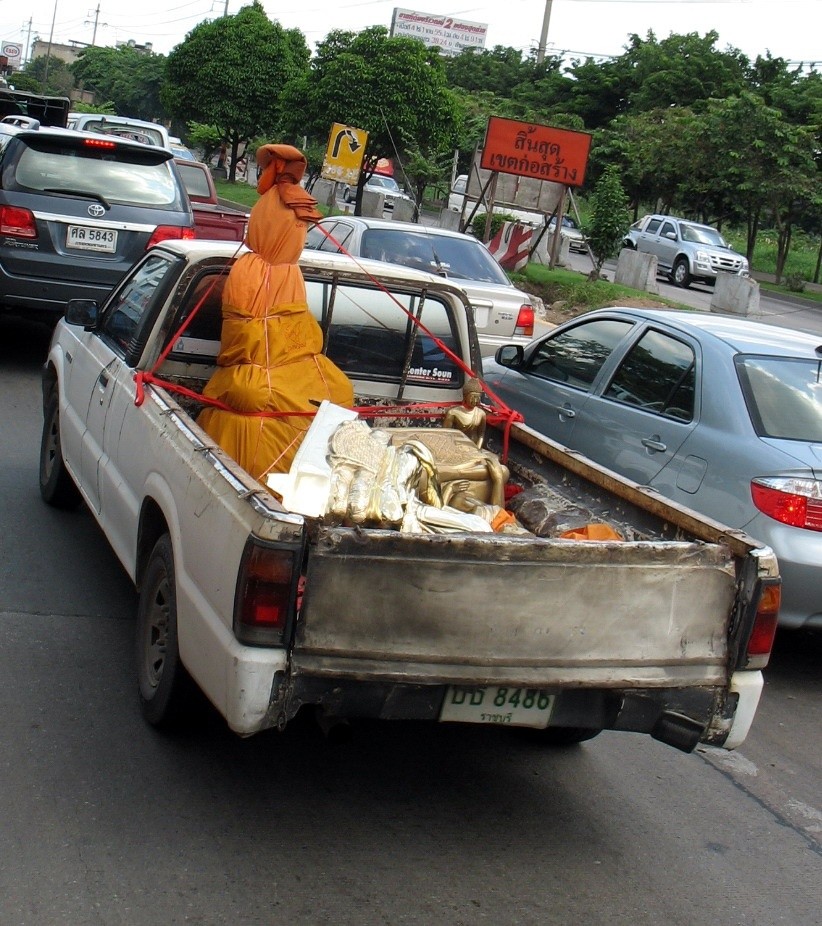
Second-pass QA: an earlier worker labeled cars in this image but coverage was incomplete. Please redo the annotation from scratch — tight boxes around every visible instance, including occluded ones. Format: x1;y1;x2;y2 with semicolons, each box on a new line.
546;216;591;254
297;217;541;370
480;305;822;645
342;173;410;208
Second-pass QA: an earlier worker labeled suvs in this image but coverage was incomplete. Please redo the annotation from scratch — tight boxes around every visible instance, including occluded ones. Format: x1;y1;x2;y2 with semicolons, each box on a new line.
0;82;251;339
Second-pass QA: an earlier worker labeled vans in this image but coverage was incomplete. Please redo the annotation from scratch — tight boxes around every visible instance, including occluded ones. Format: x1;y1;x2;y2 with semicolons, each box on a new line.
448;174;547;244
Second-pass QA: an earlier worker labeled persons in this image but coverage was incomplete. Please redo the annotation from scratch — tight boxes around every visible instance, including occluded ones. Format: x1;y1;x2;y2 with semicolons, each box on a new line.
443;377;487;449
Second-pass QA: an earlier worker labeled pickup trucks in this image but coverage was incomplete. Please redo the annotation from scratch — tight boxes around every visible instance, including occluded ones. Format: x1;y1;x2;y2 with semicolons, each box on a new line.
39;239;783;758
619;214;750;288
